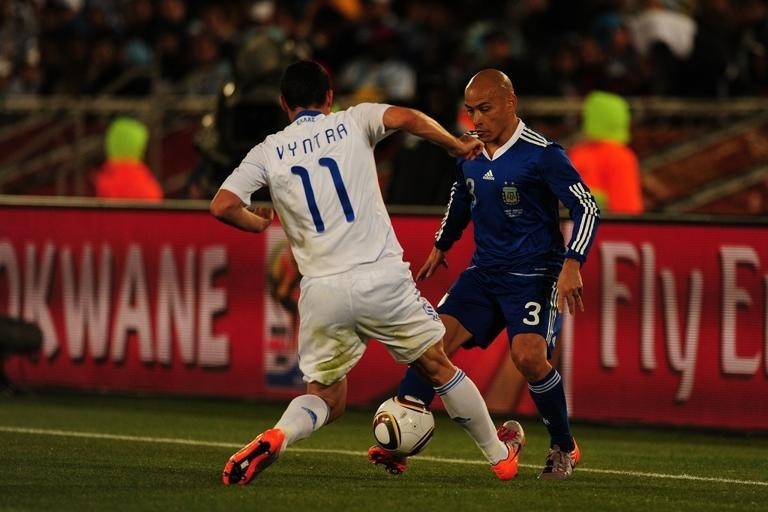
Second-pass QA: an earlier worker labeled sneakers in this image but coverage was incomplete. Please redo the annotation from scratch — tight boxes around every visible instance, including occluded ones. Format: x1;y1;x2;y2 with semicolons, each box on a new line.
223;430;289;485
491;420;525;480
368;444;407;473
538;437;581;479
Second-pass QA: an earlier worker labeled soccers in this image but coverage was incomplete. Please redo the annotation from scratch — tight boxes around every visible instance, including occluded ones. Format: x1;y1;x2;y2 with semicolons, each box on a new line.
372;395;437;457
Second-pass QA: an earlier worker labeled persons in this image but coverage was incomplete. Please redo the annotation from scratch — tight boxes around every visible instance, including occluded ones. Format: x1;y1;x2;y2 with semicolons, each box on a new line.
370;69;600;480
211;60;527;487
1;1;767;217
